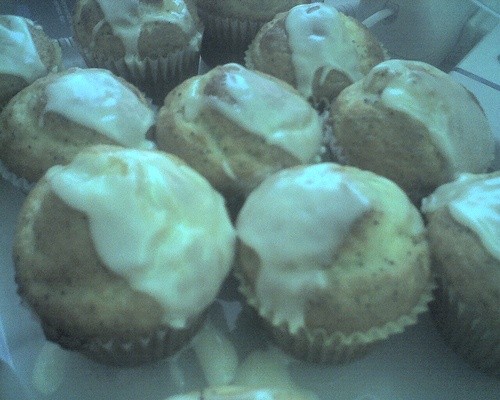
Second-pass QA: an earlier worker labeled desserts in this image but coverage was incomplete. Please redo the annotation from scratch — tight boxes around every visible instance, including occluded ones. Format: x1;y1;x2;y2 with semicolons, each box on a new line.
0;0;499;400
